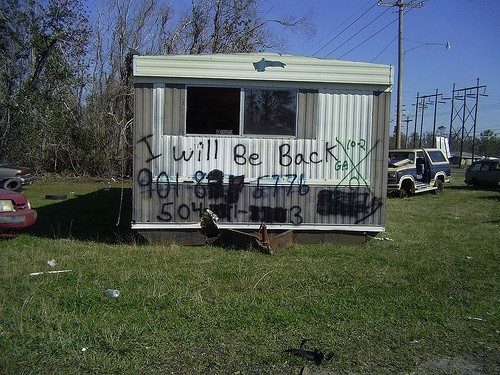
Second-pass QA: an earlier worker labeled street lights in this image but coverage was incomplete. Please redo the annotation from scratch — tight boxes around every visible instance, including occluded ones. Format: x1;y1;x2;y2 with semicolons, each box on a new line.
395;41;451;149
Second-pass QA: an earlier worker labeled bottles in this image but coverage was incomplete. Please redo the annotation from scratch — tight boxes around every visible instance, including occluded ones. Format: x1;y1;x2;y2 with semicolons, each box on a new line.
103;189;110;191
104;289;119;298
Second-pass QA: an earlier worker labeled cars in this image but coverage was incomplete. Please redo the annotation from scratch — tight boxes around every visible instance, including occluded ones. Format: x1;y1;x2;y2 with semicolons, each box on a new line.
0;165;37;238
463;158;500;191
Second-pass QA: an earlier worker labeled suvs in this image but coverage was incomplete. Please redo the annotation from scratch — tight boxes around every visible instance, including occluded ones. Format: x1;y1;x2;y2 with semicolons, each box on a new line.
388;148;452;198
